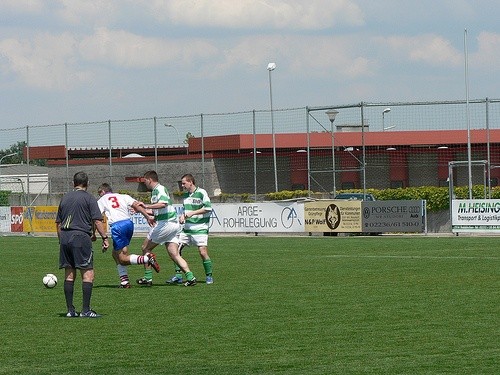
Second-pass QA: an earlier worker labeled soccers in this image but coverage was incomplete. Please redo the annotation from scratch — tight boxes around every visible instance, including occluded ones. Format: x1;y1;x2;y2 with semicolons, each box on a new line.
42;273;58;288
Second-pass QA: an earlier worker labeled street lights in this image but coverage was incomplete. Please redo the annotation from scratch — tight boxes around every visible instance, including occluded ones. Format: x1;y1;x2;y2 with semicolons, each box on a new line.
325;110;339;199
165;123;180;148
267;63;278;192
382;108;391;132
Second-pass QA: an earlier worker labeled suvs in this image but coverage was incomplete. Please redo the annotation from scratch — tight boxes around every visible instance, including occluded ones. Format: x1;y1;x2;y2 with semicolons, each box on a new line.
323;193;382;236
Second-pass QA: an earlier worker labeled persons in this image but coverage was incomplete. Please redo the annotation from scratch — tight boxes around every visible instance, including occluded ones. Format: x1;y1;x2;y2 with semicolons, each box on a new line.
166;173;213;285
128;170;197;287
55;171;110;318
90;182;160;289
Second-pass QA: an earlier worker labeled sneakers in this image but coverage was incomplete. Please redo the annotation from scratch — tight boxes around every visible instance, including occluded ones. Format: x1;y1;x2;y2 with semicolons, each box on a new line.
80;309;101;318
118;282;131;289
136;278;153;286
206;276;213;285
166;276;183;284
66;312;78;317
184;277;197;287
147;253;160;273
